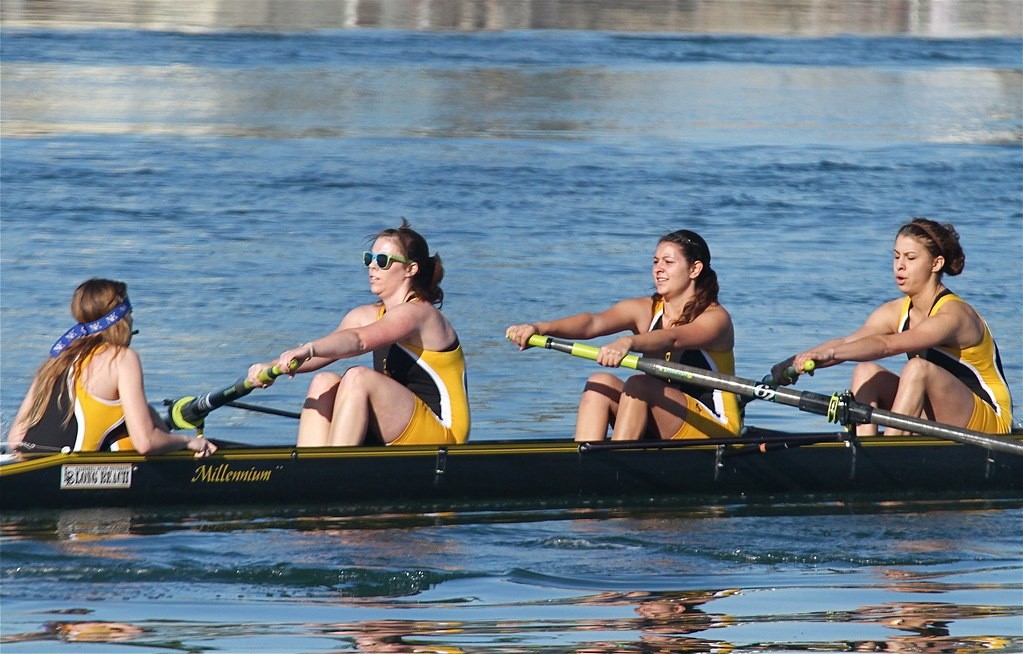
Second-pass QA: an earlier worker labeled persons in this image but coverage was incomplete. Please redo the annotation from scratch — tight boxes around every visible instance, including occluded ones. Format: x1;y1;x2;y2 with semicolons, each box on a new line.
6;278;217;458
247;216;471;447
505;228;740;440
769;216;1013;436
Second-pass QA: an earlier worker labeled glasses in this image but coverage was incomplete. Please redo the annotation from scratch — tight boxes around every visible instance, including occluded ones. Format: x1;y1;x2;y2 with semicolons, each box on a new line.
362;251;416;270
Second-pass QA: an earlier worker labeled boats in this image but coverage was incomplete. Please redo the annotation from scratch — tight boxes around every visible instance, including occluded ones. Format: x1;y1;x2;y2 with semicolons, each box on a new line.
0;430;1023;531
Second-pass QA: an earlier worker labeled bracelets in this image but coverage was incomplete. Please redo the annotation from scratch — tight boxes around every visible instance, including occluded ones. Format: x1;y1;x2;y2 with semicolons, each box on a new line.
299;343;313;362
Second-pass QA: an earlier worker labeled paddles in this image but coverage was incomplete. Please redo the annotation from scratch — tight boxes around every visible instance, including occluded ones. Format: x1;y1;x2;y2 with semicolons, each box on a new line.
735;359;815;406
146;358;299;433
506;331;1023;459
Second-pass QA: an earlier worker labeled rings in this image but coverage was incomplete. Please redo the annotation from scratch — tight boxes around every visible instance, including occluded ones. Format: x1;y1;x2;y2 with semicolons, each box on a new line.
206;449;210;452
